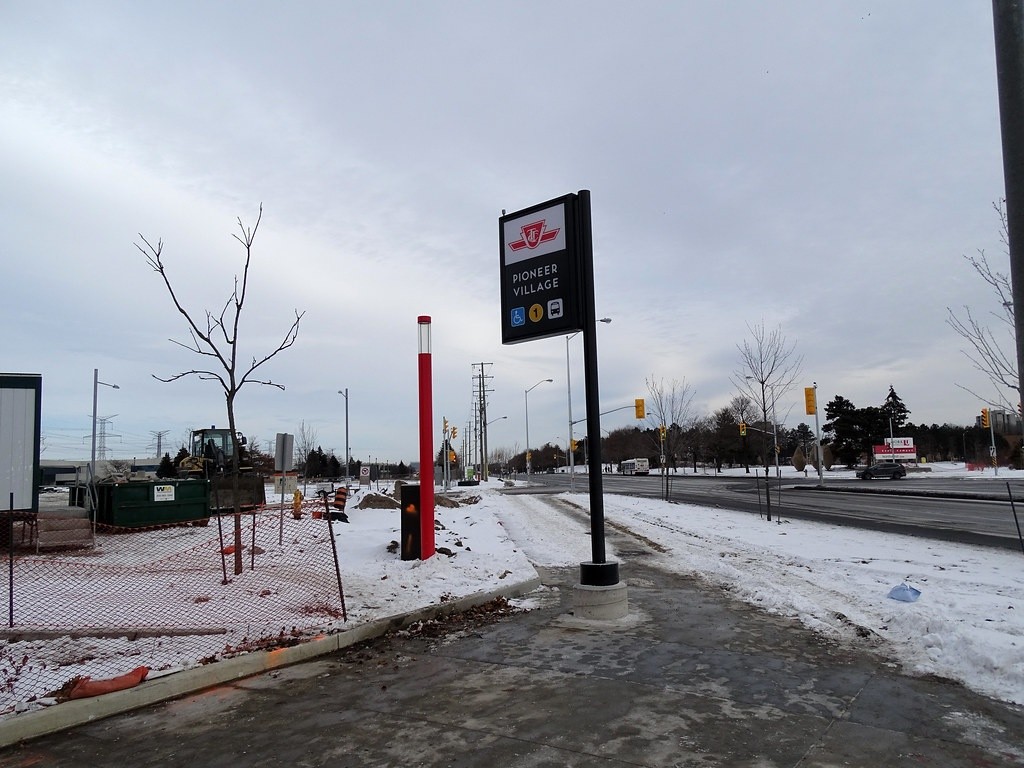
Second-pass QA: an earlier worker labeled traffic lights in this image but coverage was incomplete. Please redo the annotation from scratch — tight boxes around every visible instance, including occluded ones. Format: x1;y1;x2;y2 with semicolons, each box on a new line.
452;426;458;439
740;424;746;435
981;408;990;428
1017;402;1022;417
443;419;449;433
570;438;578;452
660;426;667;441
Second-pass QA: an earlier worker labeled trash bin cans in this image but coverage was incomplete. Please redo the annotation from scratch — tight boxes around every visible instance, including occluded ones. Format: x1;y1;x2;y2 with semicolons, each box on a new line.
70;480;210;528
921;457;926;463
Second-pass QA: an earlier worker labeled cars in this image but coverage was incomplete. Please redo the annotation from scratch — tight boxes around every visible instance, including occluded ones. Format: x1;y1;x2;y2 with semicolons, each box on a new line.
856;462;906;481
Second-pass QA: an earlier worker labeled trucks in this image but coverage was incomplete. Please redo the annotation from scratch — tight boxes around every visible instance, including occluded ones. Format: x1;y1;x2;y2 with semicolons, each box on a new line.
621;458;650;476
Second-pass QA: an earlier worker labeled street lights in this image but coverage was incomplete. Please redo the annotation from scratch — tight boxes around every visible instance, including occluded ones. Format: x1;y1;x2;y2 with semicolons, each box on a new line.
474;416;508;479
565;316;613;492
90;368;120;487
557;436;568;474
573;431;587;475
962;431;969;463
746;375;780;477
647;412;664;474
525;378;554;483
338;388;349;488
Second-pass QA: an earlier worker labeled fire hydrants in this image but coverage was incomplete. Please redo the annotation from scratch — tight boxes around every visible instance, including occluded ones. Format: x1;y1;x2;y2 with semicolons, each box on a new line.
292;489;304;520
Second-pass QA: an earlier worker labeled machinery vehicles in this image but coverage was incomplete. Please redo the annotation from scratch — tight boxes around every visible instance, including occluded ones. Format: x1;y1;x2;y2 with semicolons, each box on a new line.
176;424;248;479
